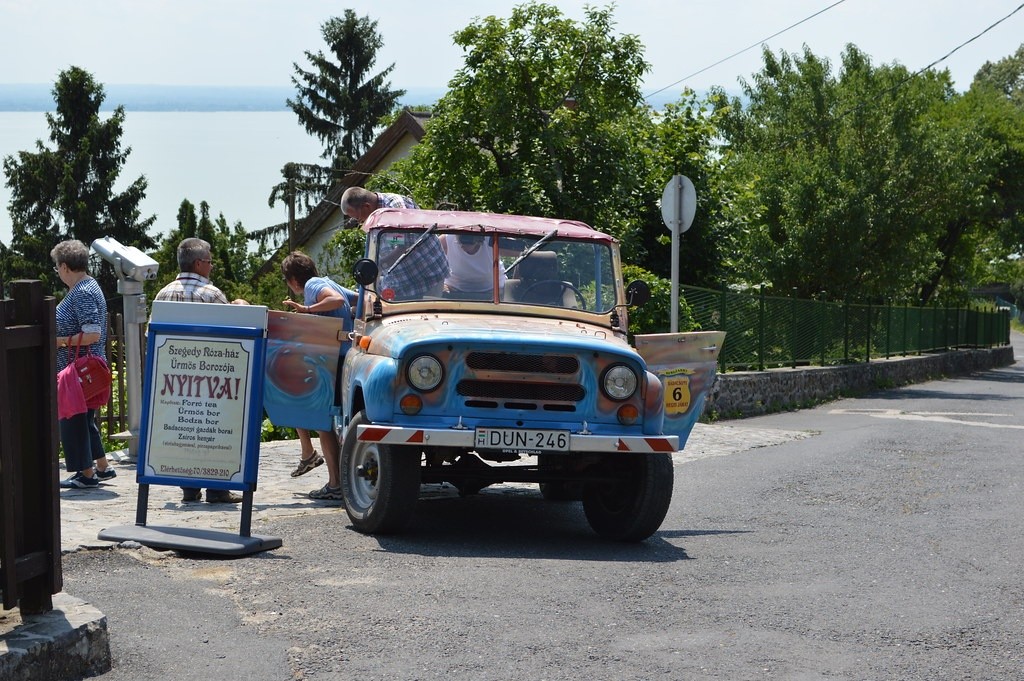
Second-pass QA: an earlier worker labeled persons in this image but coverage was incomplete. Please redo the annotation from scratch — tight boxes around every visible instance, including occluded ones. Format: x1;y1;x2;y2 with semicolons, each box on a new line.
340;186;452;300
143;238;251;504
437;231;530;302
278;251;360;500
49;240;117;488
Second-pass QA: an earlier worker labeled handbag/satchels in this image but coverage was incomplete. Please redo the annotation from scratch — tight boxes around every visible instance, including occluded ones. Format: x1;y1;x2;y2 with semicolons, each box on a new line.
67;332;111;409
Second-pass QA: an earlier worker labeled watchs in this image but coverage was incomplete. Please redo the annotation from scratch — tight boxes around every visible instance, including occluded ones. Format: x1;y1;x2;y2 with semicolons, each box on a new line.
61;337;67;348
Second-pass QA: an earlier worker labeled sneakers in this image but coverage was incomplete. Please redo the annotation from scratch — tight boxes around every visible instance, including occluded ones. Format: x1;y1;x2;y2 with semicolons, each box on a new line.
309;484;342;499
94;466;117;481
291;450;324;477
59;472;98;488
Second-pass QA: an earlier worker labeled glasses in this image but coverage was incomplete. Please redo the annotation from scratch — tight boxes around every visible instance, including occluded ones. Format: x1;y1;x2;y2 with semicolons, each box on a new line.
53;266;62;272
199;258;212;264
461;240;476;245
355;208;362;221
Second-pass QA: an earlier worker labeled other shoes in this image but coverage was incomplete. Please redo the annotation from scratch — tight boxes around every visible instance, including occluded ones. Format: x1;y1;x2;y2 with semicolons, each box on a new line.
206;492;243;503
182;493;202;503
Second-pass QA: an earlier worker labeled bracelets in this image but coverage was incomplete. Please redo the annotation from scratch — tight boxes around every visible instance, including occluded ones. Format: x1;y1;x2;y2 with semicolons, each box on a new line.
307;305;311;313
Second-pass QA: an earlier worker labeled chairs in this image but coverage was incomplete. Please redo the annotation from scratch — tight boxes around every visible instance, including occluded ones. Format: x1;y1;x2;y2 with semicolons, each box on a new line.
503;250;578;307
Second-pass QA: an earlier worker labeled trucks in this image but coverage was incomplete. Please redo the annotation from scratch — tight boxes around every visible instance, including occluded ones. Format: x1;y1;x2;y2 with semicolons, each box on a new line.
265;206;728;546
